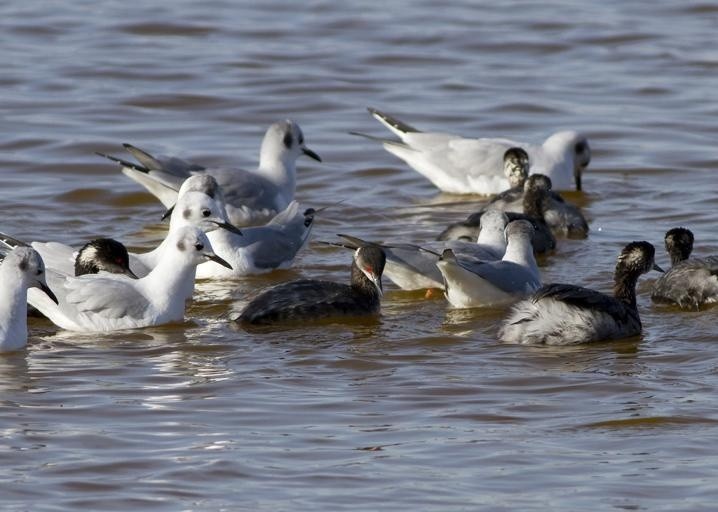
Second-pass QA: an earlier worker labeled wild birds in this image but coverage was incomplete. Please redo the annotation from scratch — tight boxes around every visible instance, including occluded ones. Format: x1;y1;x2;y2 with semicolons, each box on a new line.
0;246;59;353
437;174;556;258
93;118;323;227
159;174;329;276
652;224;718;318
0;228;234;333
436;219;543;311
223;244;388;326
493;148;590;242
349;104;593;196
73;239;139;280
0;192;243;280
494;240;666;347
319;208;511;292
